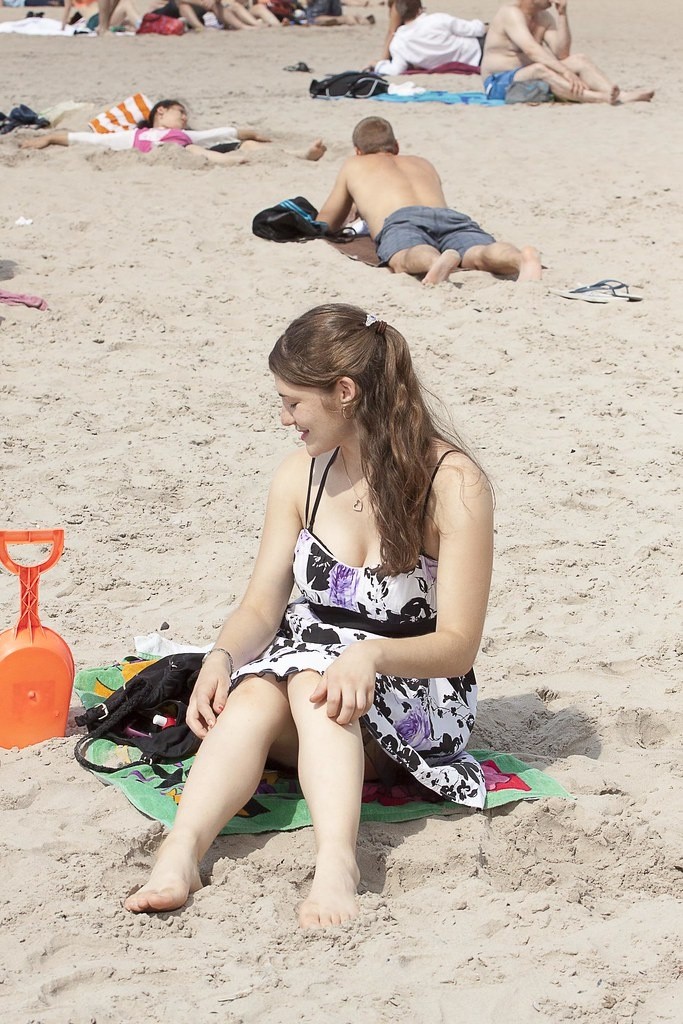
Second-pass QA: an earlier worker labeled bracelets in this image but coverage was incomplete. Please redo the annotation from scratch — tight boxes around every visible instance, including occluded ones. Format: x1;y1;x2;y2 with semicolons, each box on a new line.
201;647;234;676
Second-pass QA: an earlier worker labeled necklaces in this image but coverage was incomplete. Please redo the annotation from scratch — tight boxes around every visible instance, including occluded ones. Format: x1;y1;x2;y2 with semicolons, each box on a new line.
340;447;367;512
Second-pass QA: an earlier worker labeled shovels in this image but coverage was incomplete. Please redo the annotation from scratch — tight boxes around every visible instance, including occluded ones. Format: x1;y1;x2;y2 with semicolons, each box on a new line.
0;529;75;751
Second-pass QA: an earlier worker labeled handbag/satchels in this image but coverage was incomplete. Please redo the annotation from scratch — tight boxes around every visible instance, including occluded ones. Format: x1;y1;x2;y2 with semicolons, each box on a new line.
74;653;207;773
309;71;389;98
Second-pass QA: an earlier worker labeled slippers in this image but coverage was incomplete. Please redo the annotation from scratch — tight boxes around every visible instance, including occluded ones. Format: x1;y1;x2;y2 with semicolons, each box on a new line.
554;279;643;303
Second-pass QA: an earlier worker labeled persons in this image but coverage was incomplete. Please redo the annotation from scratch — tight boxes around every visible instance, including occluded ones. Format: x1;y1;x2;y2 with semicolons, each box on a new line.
60;0;654;105
123;302;494;930
23;99;327;163
316;116;543;286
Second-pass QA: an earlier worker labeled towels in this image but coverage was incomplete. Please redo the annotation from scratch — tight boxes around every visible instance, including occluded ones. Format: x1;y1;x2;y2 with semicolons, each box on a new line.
74;652;578;840
369;90;507;106
321;227;384;267
88;92;155;134
0;16;133;36
